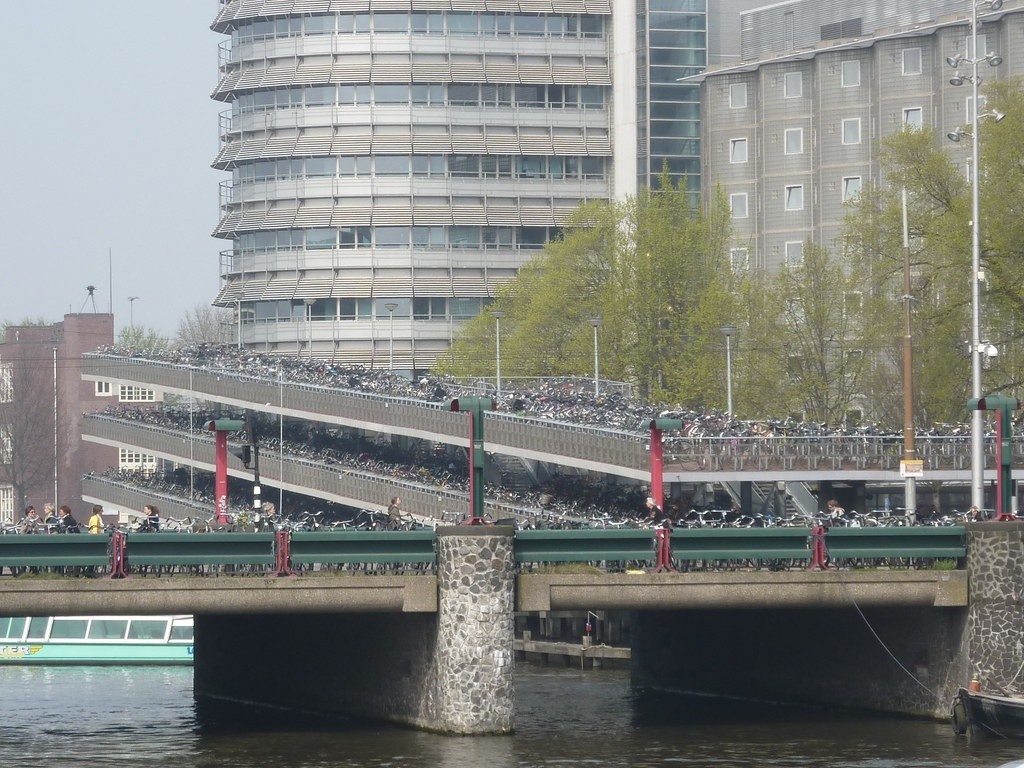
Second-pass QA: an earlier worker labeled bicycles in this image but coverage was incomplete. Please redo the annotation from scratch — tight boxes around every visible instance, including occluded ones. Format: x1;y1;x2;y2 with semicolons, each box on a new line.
1;335;1024;571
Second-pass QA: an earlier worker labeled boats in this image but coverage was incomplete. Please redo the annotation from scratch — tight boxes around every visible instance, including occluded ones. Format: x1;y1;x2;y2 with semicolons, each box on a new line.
0;616;193;665
950;689;1024;739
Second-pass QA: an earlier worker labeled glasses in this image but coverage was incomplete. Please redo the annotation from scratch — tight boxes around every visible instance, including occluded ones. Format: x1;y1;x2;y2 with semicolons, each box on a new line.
28;511;35;513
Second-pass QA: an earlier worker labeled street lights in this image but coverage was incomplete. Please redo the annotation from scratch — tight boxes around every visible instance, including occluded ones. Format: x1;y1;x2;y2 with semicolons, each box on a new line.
719;327;740;415
232;291;245;348
588;316;606;395
490;310;506;395
945;0;1005;509
305;299;316;357
385;303;399;370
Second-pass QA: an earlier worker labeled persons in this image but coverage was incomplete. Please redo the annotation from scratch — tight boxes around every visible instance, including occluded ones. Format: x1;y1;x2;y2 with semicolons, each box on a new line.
387;497;402;531
513;393;523;411
645;496;671;527
89;505;105;534
916;501;983;526
432;384;446;402
349;373;359;388
827;500;846;526
19;502;80;533
143;505;159;532
